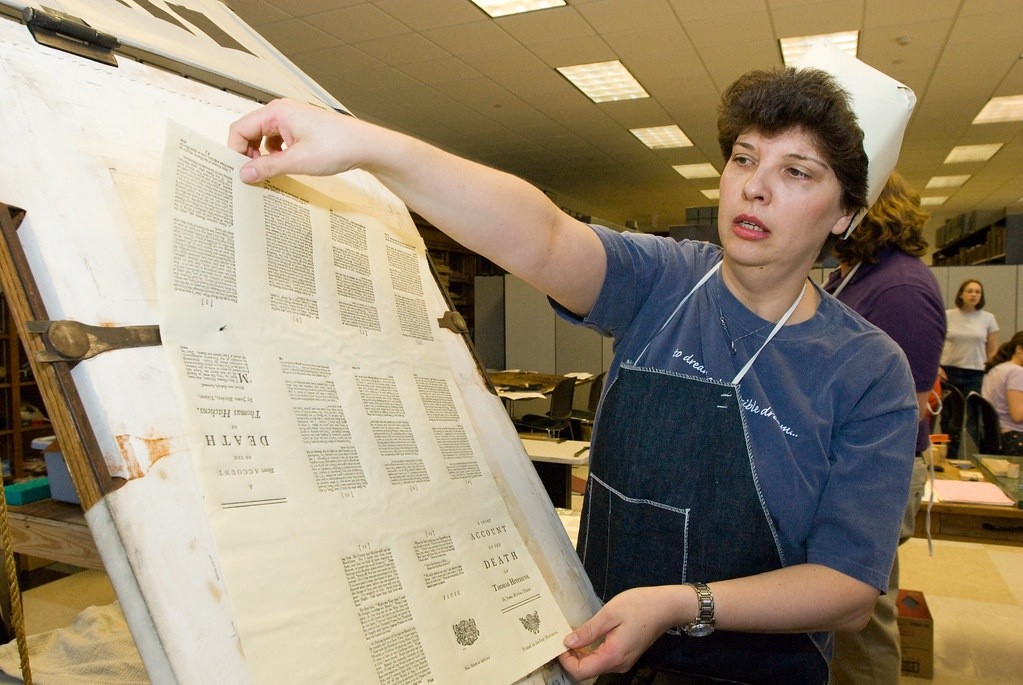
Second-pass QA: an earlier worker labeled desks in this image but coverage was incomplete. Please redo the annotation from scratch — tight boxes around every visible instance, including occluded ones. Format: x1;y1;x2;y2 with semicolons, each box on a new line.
897;538;1023;685
519;433;591;510
490;369;593;402
914;457;1021;518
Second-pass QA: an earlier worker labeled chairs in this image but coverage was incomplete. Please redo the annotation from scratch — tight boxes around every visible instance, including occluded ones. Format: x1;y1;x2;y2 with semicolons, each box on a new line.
545;370;607;436
966;390;1000;454
521;376;578;439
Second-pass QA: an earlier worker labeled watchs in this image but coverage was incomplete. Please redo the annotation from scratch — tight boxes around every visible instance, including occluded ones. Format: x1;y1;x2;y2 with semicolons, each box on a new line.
681;582;717;637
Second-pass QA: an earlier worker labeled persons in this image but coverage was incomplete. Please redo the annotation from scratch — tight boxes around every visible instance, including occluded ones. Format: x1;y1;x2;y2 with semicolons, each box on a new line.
823;170;948;685
226;67;919;685
936;279;1000;460
981;331;1023;456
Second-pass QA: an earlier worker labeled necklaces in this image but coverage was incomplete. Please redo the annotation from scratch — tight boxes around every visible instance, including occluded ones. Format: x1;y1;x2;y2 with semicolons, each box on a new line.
717;268;794;356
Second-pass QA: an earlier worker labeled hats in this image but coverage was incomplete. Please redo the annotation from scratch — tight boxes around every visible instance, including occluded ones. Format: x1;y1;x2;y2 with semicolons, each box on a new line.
788;39;918;241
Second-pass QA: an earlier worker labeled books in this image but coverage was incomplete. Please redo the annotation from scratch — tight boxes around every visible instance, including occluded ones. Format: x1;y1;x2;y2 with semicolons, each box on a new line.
981;458;1011;476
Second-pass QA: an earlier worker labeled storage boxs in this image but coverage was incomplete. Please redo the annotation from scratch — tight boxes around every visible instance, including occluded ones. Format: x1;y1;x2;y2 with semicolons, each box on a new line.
895;589;934;679
